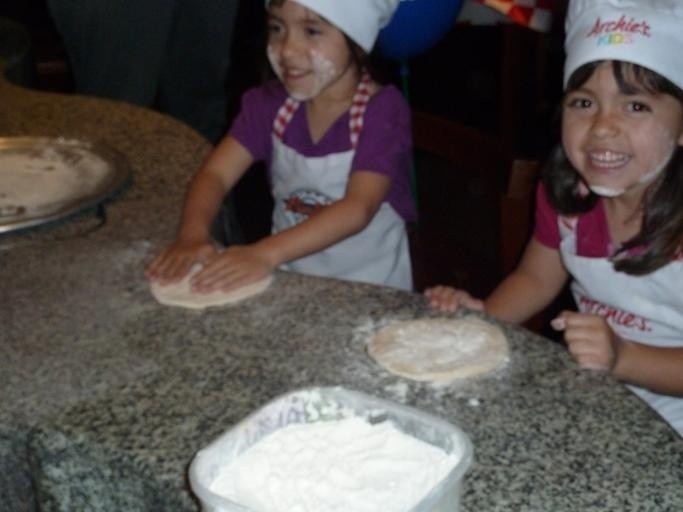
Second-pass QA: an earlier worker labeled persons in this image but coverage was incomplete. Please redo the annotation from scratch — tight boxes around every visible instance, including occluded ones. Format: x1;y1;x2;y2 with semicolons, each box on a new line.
142;0;417;294
424;0;683;438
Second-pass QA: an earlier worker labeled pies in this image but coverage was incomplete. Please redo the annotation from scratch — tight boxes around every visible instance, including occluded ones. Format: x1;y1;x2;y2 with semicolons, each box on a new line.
367;315;508;382
150;250;275;308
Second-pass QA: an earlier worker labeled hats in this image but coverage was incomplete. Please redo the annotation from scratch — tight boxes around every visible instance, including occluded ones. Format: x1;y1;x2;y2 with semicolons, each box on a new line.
561;1;682;95
264;0;402;58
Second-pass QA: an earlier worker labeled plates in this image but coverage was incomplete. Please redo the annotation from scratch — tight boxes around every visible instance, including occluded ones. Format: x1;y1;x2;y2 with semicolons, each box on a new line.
0;135;130;234
